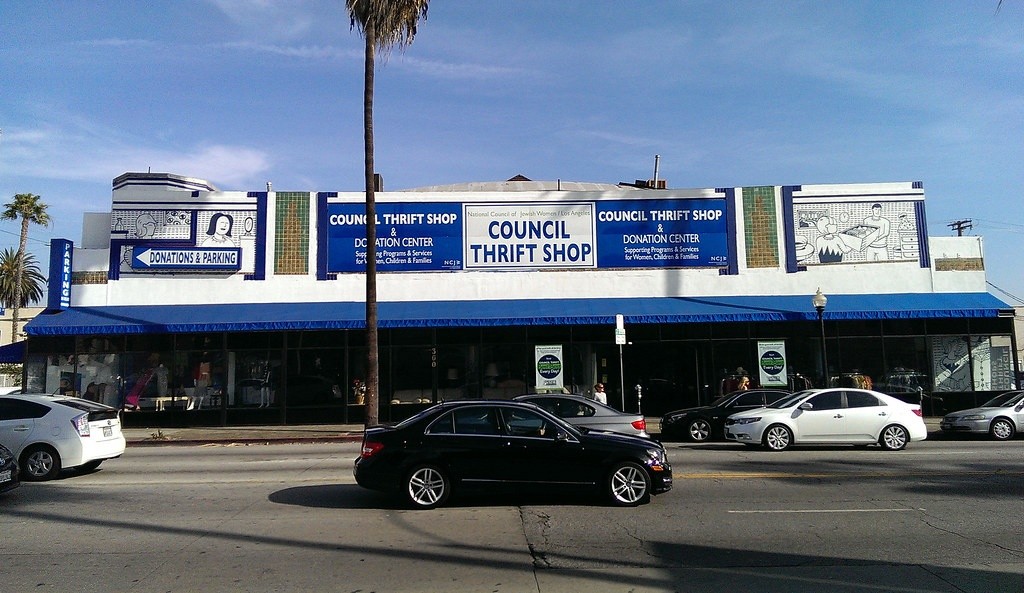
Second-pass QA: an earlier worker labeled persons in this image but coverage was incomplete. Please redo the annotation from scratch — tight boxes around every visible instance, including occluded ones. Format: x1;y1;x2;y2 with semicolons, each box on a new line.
152;363;169;411
186;357;210;410
594;383;607;405
738;376;750;391
258;360;271;408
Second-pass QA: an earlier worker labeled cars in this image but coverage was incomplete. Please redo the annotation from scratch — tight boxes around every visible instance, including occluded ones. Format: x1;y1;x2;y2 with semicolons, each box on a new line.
0;390;126;492
474;392;653;441
352;399;672;508
939;389;1024;441
658;388;928;451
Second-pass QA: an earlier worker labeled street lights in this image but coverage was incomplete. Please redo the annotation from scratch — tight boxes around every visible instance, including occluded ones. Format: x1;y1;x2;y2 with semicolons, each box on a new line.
810;286;829;388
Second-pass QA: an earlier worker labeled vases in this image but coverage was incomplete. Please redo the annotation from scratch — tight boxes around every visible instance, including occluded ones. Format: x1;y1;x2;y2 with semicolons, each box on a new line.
356;395;364;404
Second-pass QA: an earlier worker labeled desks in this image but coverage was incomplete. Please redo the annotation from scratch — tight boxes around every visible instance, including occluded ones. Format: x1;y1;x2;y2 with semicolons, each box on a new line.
155;395;194;411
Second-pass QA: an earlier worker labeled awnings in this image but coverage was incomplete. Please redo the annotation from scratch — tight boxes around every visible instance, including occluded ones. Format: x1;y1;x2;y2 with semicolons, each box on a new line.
22;291;1015;336
0;339;45;363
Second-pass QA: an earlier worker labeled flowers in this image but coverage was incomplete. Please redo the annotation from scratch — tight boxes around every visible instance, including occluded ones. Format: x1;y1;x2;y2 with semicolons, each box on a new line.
352;380;366;396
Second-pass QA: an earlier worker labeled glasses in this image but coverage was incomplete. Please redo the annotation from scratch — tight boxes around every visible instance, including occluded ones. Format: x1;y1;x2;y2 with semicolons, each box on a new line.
599;387;604;389
